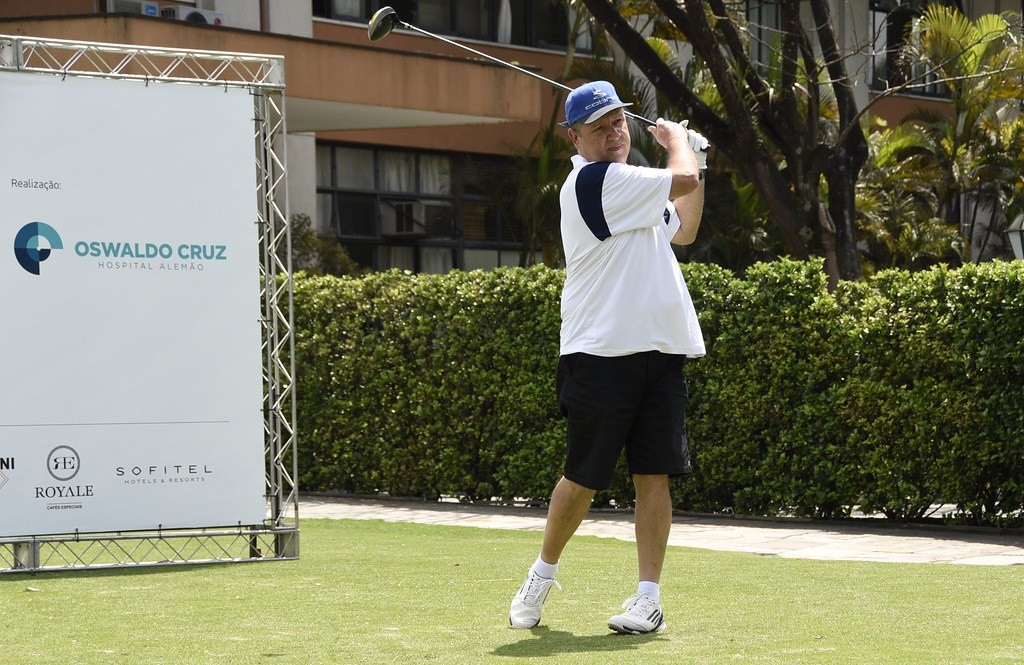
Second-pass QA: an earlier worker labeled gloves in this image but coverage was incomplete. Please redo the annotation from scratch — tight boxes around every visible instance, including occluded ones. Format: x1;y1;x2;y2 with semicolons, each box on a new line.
678;119;709;170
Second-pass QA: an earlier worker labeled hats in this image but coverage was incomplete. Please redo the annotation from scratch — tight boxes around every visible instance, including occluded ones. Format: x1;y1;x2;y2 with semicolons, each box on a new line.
556;80;634;128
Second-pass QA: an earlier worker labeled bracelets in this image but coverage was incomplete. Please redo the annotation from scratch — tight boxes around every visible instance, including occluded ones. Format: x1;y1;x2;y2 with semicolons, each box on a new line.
698;171;706;181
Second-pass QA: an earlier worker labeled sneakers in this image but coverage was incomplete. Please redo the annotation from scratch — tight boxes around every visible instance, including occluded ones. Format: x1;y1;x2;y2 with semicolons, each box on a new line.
607;590;666;634
509;564;563;629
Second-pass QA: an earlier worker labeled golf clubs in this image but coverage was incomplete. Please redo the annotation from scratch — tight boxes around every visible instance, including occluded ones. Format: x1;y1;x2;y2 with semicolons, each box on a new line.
366;6;711;155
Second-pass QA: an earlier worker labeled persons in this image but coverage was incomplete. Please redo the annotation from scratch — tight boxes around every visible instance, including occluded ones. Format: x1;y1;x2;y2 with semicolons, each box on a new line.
510;81;708;634
